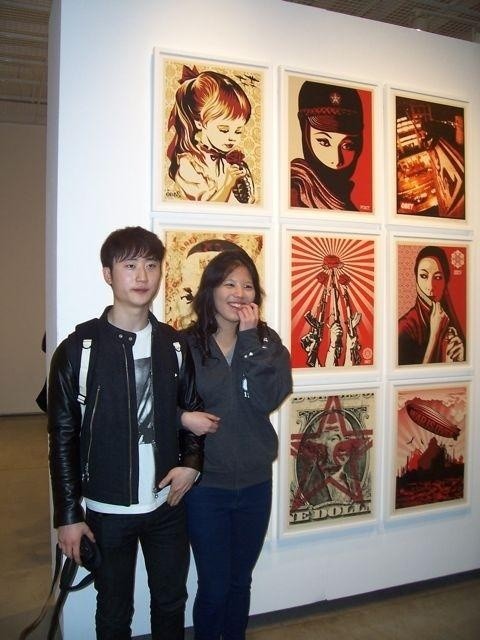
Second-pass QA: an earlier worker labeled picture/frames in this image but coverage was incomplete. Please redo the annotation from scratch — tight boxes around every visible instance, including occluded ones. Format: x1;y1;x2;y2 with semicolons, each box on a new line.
152;224;275;333
383;232;475;381
384;375;474;533
151;46;275;232
386;86;474;233
275;60;386;228
277;223;383;387
275;384;384;545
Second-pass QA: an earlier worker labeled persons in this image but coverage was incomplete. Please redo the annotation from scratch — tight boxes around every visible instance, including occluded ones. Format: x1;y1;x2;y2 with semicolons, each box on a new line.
168;65;251;202
398;246;466;364
181;249;293;639
291;81;365;211
300;323;361;367
49;227;204;639
302;413;363;506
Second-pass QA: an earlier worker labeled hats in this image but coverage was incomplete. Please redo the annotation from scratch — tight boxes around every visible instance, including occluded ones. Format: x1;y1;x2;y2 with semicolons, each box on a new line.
296;79;365;136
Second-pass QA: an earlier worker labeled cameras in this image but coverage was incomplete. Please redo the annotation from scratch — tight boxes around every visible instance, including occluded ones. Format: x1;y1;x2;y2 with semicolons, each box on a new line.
80;535;93;564
